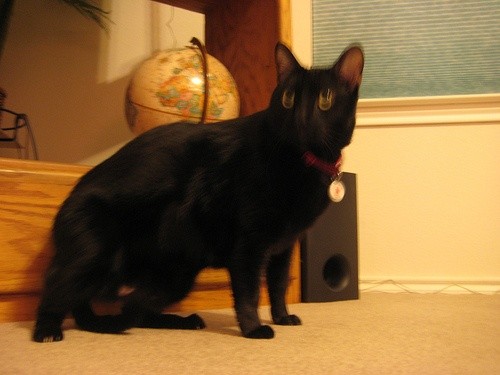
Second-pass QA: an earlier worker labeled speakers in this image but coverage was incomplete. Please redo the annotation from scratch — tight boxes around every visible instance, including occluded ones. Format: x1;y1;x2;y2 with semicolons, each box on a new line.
297;170;359;304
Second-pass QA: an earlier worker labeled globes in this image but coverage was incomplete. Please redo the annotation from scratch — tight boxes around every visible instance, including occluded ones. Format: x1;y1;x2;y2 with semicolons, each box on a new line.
123;37;241;138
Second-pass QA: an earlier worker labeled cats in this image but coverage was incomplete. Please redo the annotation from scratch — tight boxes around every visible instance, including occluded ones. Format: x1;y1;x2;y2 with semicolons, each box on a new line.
30;41;364;343
